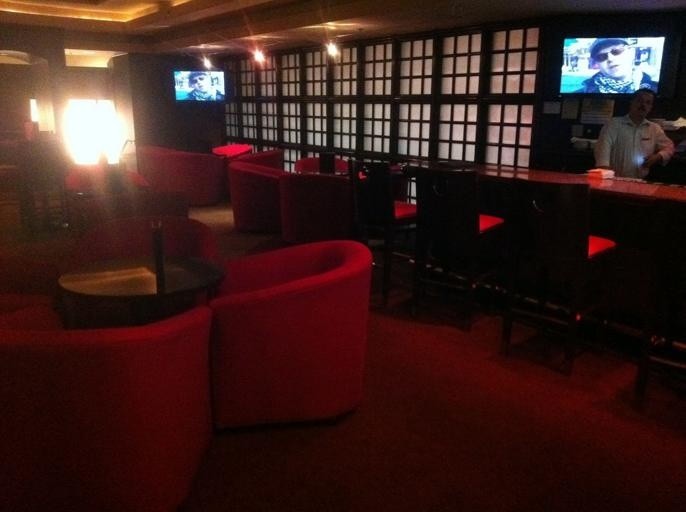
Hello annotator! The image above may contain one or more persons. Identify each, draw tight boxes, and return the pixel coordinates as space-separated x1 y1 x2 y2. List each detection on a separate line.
591 87 674 179
183 72 224 101
574 37 658 94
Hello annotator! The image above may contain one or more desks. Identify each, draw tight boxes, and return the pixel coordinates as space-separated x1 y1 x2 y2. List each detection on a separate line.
55 255 225 317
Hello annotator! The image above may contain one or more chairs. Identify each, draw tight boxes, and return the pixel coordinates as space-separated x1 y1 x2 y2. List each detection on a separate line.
0 301 215 512
206 235 376 431
401 165 497 331
493 175 620 375
69 216 224 262
136 145 399 246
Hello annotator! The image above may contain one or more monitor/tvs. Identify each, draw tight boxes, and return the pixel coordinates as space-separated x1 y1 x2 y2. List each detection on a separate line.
550 29 682 100
168 65 233 106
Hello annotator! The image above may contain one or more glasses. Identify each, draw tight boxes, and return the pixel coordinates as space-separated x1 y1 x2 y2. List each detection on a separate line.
190 77 203 84
594 45 628 62
